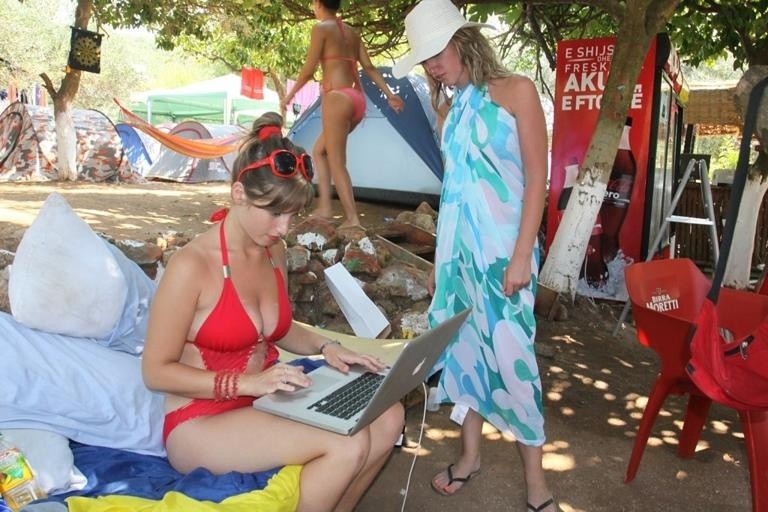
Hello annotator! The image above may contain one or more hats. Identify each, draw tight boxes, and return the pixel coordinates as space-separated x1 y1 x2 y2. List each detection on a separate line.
391 0 495 80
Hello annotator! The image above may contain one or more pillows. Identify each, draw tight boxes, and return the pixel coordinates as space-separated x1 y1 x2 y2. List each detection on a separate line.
6 189 159 343
0 425 89 500
1 308 167 458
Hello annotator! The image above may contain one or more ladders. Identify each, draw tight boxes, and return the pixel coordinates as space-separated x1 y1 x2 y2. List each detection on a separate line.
612 159 720 338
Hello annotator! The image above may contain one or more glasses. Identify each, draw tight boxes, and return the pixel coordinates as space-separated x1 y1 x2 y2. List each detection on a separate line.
236 149 314 181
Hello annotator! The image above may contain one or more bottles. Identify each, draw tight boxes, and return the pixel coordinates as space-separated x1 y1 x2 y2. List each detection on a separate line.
600 116 637 266
0 435 47 512
554 155 610 292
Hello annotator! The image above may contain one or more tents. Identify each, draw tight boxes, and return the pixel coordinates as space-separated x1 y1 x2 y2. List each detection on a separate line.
0 98 248 185
286 65 444 211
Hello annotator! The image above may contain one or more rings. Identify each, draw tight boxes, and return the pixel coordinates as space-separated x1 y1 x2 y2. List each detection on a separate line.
283 367 288 374
284 375 288 383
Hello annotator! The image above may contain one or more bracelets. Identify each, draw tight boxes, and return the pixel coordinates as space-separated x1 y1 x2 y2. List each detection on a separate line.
232 374 240 400
225 373 232 400
214 373 223 402
319 340 342 351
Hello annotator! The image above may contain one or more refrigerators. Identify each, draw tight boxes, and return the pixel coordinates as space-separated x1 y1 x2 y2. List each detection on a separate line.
546 32 691 300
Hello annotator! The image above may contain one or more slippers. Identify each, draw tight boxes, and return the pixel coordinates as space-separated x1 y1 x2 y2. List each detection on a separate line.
430 464 480 496
526 499 552 512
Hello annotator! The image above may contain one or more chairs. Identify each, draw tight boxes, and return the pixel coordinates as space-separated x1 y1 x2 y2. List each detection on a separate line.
621 257 768 511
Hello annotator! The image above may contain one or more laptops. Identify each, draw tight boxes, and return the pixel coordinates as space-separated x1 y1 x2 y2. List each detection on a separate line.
253 306 472 437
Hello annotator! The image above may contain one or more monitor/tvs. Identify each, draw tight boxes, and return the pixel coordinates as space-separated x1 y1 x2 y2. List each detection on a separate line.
680 154 710 180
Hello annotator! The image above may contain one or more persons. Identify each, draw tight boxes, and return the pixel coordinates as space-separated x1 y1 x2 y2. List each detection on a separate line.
142 112 405 512
281 1 404 229
389 0 560 512
